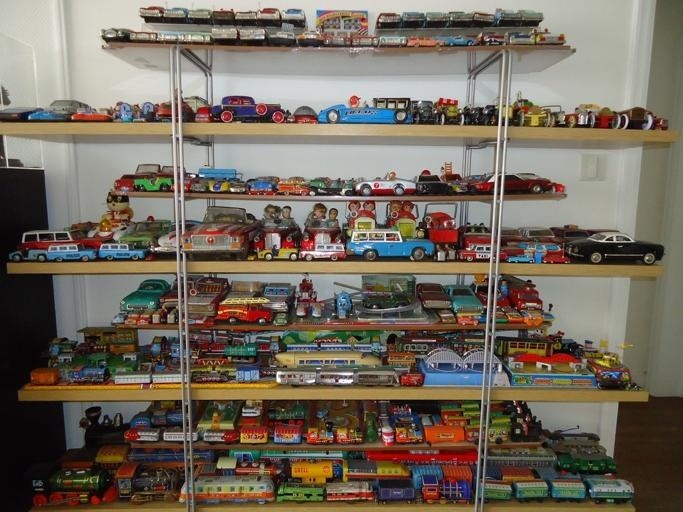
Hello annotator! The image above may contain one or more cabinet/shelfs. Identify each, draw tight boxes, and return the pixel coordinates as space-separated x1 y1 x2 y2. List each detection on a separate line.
3 31 679 512
